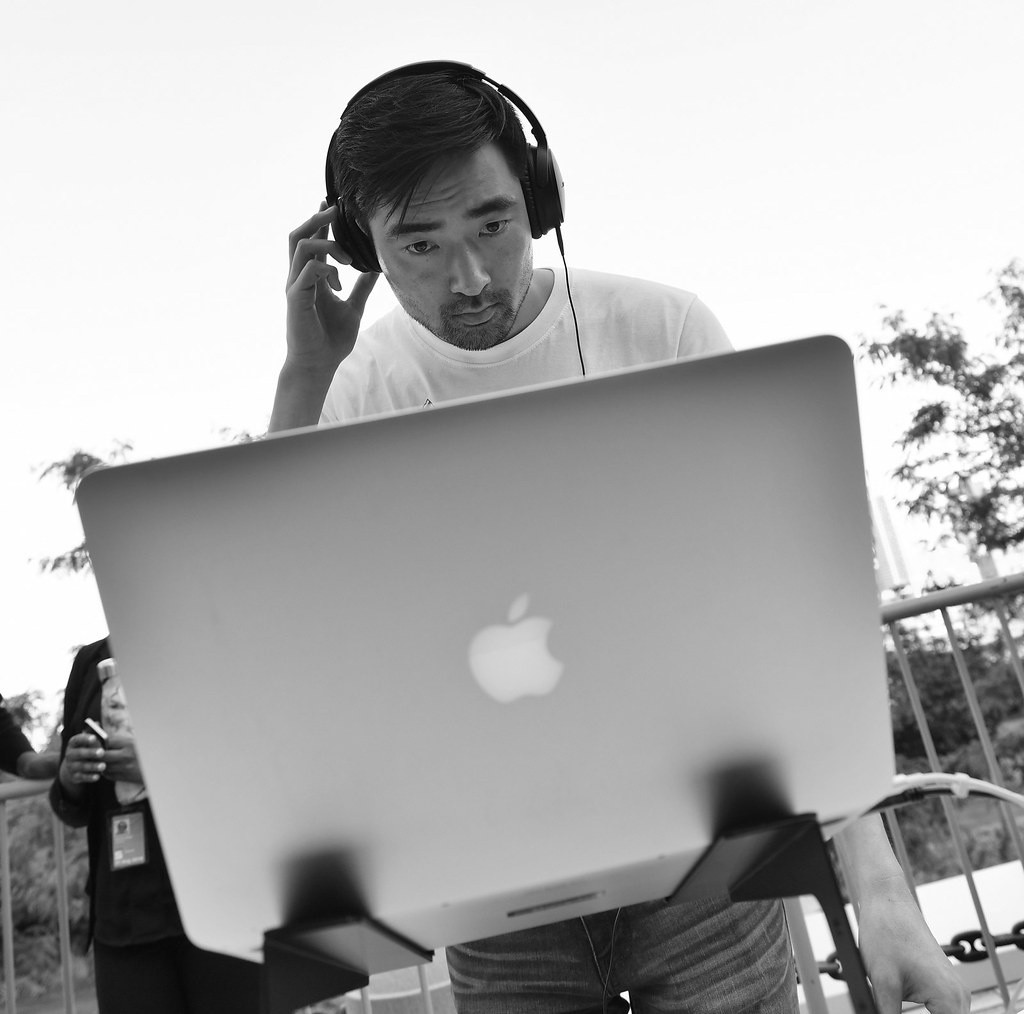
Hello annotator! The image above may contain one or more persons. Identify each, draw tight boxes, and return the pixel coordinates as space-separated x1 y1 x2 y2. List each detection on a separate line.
0 636 265 1014
270 60 972 1014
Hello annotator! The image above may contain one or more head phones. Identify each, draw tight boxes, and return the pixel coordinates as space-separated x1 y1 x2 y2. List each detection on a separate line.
325 58 563 271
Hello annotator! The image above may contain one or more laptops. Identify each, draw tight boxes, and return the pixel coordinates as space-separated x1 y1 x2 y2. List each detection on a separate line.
74 333 895 961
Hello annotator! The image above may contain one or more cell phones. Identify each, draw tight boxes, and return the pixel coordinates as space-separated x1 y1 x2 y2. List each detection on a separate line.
83 717 109 749
